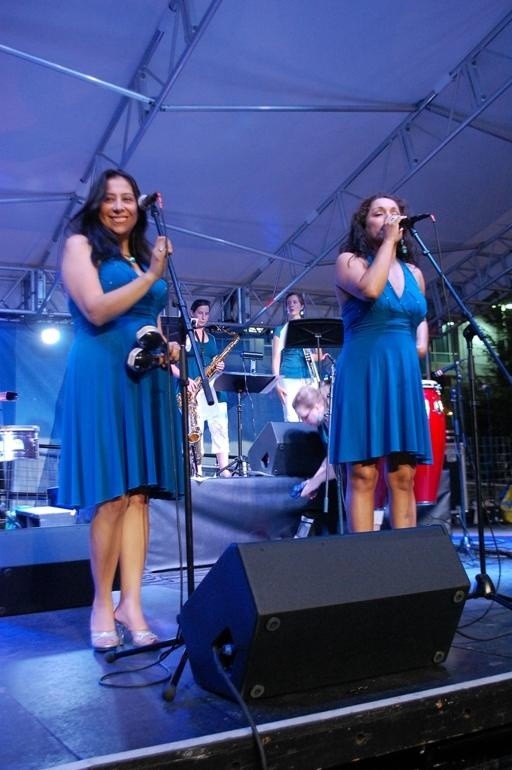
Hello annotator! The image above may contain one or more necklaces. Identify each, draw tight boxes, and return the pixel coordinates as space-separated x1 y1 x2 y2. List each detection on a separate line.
121 251 137 263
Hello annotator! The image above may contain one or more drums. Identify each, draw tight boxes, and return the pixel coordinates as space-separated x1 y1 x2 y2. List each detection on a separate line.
342 379 446 509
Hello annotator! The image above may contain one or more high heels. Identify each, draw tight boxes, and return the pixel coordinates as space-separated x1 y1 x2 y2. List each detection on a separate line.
90 621 159 651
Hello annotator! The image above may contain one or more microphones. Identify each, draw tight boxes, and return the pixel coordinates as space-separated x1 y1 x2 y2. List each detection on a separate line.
397 210 434 234
434 357 466 379
0 391 19 404
136 189 165 216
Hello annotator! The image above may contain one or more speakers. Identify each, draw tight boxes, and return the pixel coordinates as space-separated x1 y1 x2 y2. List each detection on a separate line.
247 418 328 477
0 522 109 618
175 522 471 705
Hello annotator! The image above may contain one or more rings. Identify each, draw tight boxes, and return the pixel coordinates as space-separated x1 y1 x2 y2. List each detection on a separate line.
158 246 163 252
390 217 397 221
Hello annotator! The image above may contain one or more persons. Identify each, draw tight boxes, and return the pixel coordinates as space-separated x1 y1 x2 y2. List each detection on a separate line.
326 189 430 534
170 299 234 480
291 385 342 502
53 166 187 653
271 292 324 423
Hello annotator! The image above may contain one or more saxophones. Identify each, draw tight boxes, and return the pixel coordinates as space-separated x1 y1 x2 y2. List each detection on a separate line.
176 325 240 443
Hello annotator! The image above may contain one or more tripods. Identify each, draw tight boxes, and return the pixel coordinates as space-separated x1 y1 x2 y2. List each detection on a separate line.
215 393 253 478
429 325 512 616
454 382 512 563
